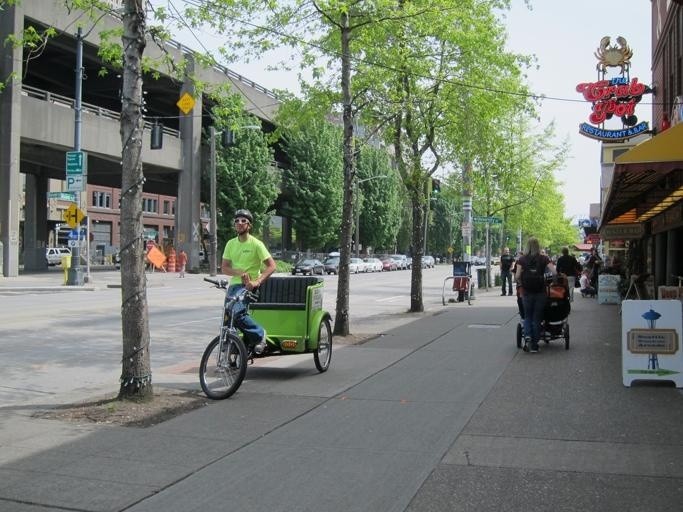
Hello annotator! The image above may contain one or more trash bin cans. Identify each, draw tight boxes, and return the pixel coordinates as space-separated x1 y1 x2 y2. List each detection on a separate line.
495 276 503 286
62 255 71 282
477 268 486 289
112 254 119 265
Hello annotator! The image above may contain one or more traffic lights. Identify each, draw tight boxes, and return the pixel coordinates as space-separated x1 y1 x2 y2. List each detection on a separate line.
433 179 440 192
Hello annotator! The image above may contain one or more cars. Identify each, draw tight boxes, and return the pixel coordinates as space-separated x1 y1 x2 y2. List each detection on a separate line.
470 256 501 266
291 255 434 276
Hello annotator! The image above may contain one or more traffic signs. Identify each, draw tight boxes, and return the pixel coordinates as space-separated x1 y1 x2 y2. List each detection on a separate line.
473 216 502 223
66 151 88 192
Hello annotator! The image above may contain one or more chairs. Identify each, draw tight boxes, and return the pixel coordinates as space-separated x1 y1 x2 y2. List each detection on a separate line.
250 276 325 336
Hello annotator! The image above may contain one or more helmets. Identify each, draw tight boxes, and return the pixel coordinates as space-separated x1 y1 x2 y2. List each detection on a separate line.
234 209 254 223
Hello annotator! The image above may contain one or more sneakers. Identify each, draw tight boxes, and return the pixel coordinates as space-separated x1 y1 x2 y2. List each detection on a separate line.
523 337 538 352
254 329 267 351
500 293 512 296
214 361 231 372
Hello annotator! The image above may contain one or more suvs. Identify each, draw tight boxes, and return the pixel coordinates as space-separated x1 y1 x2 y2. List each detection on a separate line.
45 248 71 265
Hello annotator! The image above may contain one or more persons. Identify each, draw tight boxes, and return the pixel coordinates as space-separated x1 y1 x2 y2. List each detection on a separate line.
177 249 187 278
500 247 619 298
213 209 277 374
514 237 557 354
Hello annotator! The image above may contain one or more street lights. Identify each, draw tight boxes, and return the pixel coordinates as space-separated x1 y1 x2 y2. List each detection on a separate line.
355 175 389 257
209 125 262 276
425 198 438 201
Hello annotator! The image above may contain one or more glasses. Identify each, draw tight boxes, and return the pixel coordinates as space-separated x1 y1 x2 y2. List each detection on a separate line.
235 219 249 224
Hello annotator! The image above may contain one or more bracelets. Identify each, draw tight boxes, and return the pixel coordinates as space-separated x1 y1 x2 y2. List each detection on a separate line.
255 278 260 288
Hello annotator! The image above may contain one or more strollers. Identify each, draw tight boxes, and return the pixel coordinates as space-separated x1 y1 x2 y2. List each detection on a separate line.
516 277 570 350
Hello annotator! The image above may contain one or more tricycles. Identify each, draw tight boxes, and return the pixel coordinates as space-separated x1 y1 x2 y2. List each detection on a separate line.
199 276 334 400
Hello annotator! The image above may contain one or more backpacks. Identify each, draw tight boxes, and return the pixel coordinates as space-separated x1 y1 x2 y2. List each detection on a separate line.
520 261 544 293
